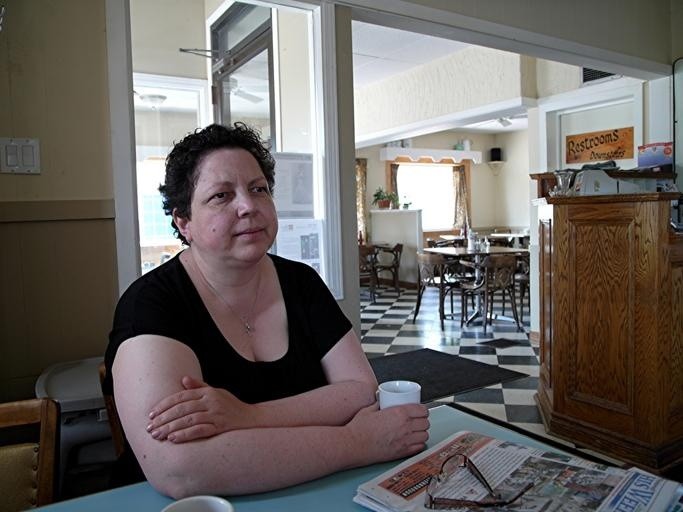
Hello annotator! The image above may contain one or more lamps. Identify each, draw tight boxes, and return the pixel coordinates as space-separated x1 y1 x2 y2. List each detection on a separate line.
138 94 167 110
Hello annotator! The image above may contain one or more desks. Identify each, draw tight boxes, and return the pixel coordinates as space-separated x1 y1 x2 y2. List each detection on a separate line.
29 399 683 512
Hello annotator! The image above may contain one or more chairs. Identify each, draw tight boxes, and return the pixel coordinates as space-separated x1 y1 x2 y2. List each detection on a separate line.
355 228 531 338
97 361 145 486
0 398 61 512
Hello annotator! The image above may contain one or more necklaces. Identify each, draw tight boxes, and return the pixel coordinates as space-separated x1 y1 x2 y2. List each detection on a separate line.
192 262 262 336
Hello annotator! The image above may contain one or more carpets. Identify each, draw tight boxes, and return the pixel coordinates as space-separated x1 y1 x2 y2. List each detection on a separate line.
367 348 533 405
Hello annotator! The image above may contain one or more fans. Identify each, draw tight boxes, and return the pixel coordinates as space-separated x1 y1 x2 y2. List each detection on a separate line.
229 77 264 104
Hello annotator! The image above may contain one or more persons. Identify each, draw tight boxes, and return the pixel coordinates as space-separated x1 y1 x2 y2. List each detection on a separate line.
103 121 430 502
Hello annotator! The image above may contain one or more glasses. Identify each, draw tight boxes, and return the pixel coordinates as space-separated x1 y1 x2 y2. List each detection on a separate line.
424 453 534 510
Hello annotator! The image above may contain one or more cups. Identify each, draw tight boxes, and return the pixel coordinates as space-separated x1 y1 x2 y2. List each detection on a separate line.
553 171 574 196
374 380 422 413
159 495 232 512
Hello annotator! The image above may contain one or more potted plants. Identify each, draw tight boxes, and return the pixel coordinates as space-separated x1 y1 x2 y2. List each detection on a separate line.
371 186 400 209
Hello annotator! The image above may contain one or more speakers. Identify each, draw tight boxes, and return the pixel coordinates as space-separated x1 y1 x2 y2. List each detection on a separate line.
490 147 502 160
